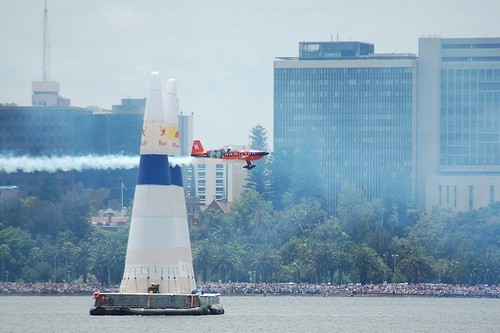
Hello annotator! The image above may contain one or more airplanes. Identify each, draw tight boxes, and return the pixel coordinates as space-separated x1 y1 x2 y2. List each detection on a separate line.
190 141 269 171
